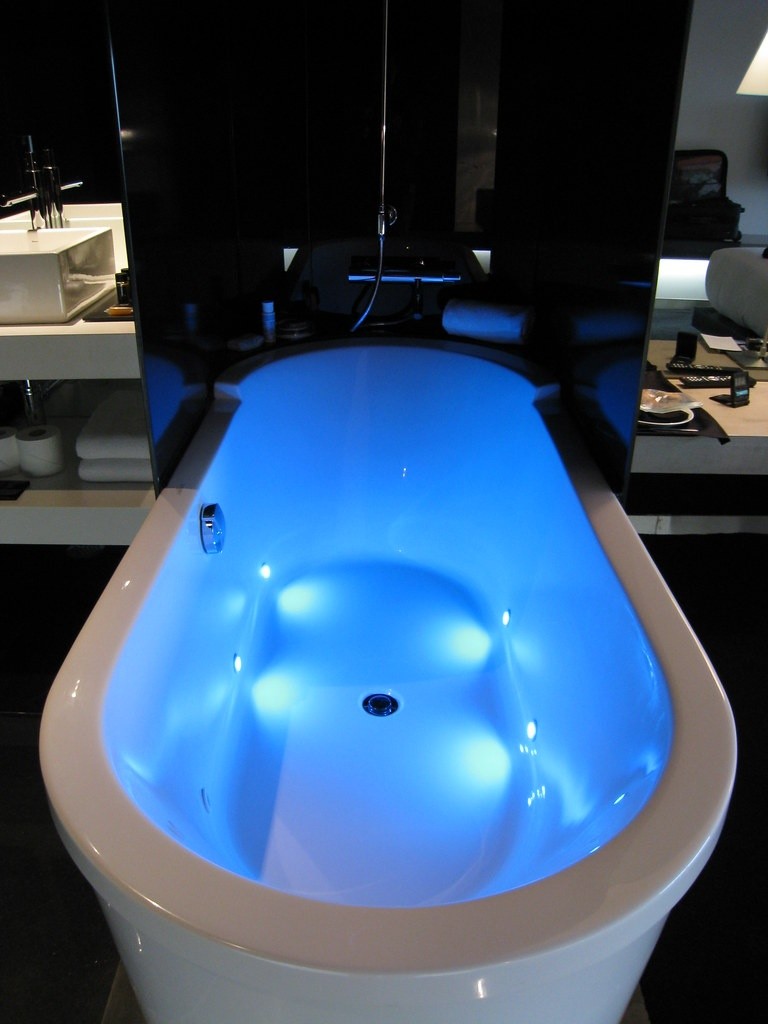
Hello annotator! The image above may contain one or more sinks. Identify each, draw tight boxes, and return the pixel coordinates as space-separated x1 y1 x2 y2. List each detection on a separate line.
0 203 128 273
0 228 116 324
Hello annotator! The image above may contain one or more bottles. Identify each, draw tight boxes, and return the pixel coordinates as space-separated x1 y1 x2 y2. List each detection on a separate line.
115 268 130 304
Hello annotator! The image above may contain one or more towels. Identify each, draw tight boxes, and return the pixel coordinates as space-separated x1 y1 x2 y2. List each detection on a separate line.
76 390 155 482
442 300 525 343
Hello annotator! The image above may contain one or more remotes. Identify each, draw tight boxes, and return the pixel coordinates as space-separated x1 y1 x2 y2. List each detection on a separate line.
680 375 756 389
665 362 743 375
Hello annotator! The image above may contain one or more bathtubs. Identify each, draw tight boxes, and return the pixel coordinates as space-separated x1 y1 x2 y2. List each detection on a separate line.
39 336 738 1024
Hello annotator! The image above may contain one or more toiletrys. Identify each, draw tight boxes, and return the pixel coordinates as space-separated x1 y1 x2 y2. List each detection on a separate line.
263 302 275 339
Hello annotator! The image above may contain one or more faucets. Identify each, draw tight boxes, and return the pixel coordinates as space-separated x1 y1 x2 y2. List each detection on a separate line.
0 162 66 228
30 161 83 226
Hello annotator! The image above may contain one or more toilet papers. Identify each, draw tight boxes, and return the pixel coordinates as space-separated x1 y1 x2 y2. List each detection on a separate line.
0 426 65 476
